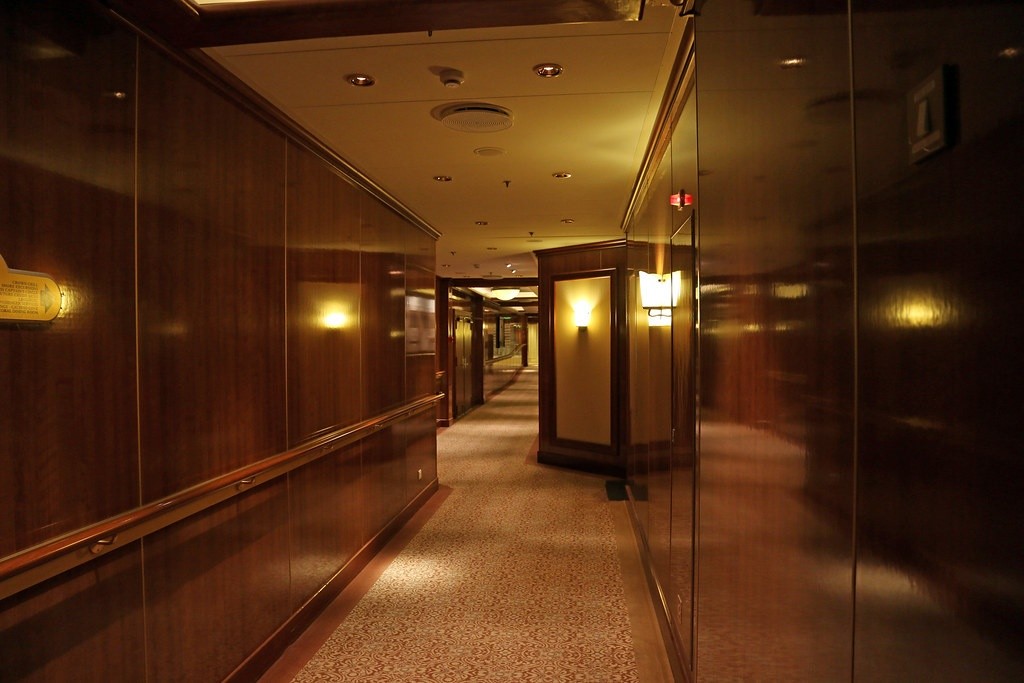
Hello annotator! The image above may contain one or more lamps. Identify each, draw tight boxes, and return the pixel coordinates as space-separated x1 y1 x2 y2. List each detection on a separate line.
638 270 662 317
